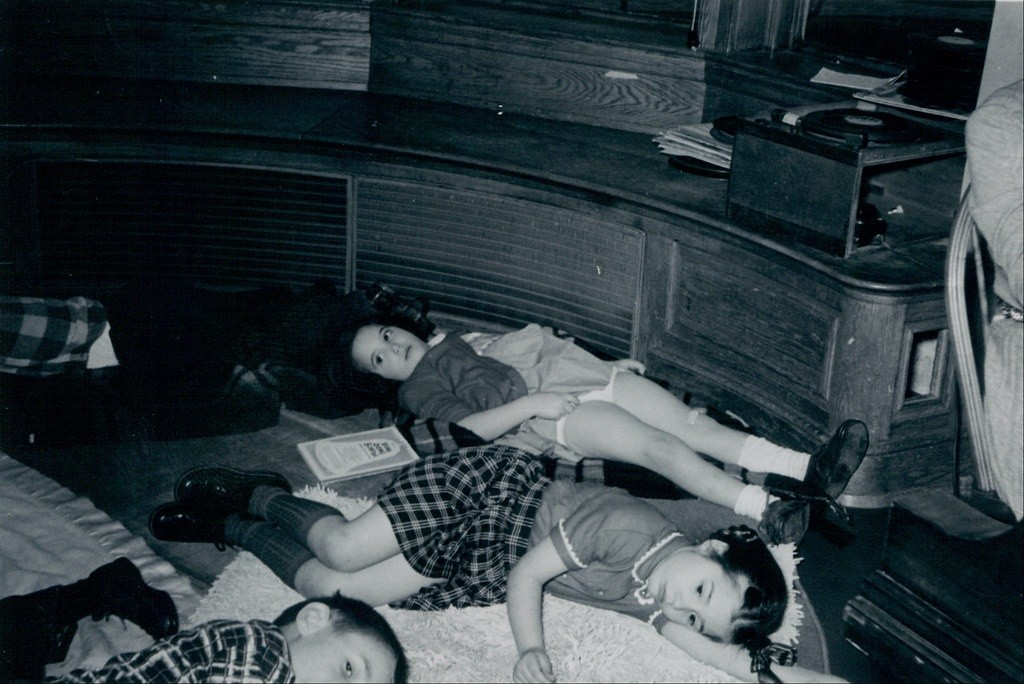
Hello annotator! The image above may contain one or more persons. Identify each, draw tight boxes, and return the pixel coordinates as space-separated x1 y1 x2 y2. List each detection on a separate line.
962 75 1024 522
318 300 869 546
47 588 413 684
145 445 849 684
0 556 180 684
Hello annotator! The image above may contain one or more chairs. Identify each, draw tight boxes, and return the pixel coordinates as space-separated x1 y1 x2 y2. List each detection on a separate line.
944 178 996 501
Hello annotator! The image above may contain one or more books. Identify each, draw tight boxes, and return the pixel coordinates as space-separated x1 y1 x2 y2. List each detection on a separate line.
654 118 736 172
297 424 421 486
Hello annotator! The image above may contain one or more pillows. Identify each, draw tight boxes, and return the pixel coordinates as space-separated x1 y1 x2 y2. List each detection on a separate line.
0 295 107 377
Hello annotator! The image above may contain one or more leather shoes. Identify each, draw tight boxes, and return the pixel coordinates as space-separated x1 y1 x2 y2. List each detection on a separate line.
148 501 239 553
174 466 292 526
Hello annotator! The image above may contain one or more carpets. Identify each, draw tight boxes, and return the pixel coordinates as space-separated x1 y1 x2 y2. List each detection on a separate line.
179 485 803 681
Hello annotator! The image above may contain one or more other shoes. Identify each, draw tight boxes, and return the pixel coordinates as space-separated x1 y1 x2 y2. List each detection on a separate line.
759 494 812 545
803 419 870 502
86 555 180 638
